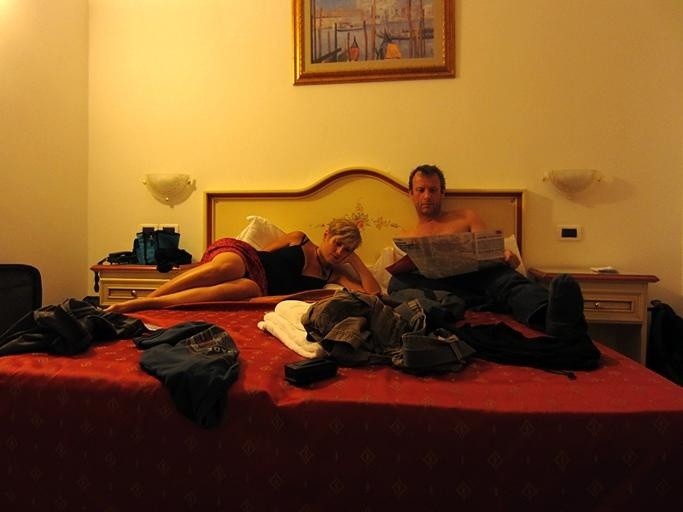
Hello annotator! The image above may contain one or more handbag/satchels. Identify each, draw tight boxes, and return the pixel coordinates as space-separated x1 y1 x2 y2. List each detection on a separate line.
135 225 181 263
647 299 683 385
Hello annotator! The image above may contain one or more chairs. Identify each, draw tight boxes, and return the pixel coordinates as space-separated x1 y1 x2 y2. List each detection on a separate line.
0 265 42 339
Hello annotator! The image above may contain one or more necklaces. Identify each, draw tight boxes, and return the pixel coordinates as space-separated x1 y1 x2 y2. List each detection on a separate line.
314 247 327 277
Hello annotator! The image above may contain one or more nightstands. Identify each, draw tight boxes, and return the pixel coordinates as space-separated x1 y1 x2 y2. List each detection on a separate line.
529 265 659 367
90 263 195 311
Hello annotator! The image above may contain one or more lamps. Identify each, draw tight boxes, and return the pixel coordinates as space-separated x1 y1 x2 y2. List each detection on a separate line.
541 169 603 199
140 172 191 201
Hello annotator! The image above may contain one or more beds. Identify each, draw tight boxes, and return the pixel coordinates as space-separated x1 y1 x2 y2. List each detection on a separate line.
0 166 683 511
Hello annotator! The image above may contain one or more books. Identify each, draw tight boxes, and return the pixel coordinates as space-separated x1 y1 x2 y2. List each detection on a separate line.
589 266 619 272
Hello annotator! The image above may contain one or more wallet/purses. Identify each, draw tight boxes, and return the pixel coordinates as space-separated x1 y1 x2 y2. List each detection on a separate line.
284 355 338 385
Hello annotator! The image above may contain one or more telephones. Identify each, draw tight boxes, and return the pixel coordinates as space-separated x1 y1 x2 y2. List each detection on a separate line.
107 250 139 265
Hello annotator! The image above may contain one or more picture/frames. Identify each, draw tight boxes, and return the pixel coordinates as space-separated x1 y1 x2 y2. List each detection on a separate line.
292 0 456 87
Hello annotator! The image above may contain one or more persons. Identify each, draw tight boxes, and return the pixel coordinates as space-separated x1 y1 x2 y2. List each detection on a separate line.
384 162 585 339
102 218 381 317
